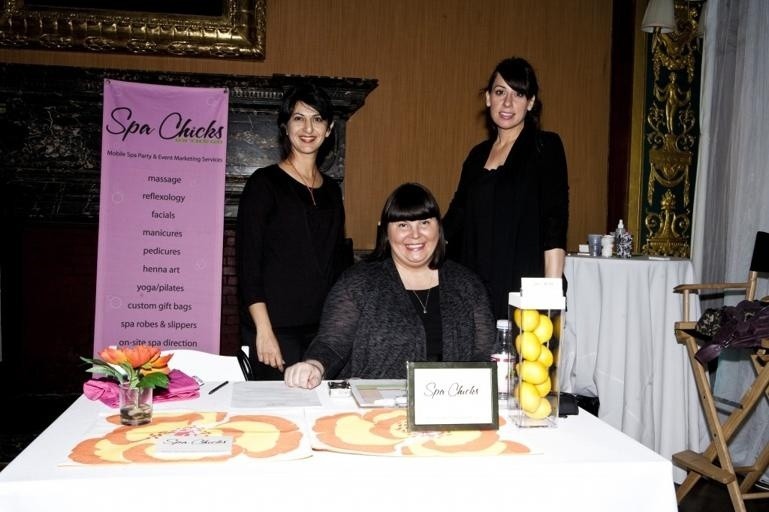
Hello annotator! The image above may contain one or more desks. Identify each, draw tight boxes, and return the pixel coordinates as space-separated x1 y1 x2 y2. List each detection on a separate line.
559 257 710 485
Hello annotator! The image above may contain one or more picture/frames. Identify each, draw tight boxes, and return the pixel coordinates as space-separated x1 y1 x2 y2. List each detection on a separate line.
0 0 266 62
406 362 497 431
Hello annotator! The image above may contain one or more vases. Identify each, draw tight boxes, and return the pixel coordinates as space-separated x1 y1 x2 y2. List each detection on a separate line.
119 387 152 425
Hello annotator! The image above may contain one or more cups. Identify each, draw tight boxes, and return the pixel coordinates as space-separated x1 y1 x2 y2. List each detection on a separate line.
120 383 154 426
588 234 602 256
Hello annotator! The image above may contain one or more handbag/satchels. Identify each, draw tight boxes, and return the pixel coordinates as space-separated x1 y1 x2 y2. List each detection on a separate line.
692 298 769 365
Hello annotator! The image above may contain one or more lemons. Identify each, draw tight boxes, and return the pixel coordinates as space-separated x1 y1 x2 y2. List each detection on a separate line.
526 398 551 419
517 360 547 384
520 332 540 360
521 310 539 332
538 343 553 368
535 376 551 397
513 308 523 331
519 382 539 412
534 313 553 342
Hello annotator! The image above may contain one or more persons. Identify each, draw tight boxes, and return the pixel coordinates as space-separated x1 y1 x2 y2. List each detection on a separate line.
284 183 495 390
438 56 569 334
235 84 344 380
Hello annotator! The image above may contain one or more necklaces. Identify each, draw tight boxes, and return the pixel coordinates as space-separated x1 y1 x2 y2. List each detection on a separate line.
397 266 434 313
287 159 318 205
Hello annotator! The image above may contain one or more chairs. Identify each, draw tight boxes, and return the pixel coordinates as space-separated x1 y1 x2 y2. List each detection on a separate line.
672 232 769 512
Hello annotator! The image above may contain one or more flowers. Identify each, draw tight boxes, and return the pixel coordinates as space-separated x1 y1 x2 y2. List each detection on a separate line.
80 345 174 388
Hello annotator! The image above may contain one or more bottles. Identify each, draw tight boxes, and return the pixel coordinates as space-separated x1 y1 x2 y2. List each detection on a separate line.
615 219 626 257
490 320 516 399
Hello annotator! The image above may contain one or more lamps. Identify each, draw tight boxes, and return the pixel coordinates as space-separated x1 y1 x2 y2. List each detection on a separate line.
642 0 681 57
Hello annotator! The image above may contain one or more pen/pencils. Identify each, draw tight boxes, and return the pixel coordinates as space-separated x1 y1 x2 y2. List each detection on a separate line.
209 381 230 395
